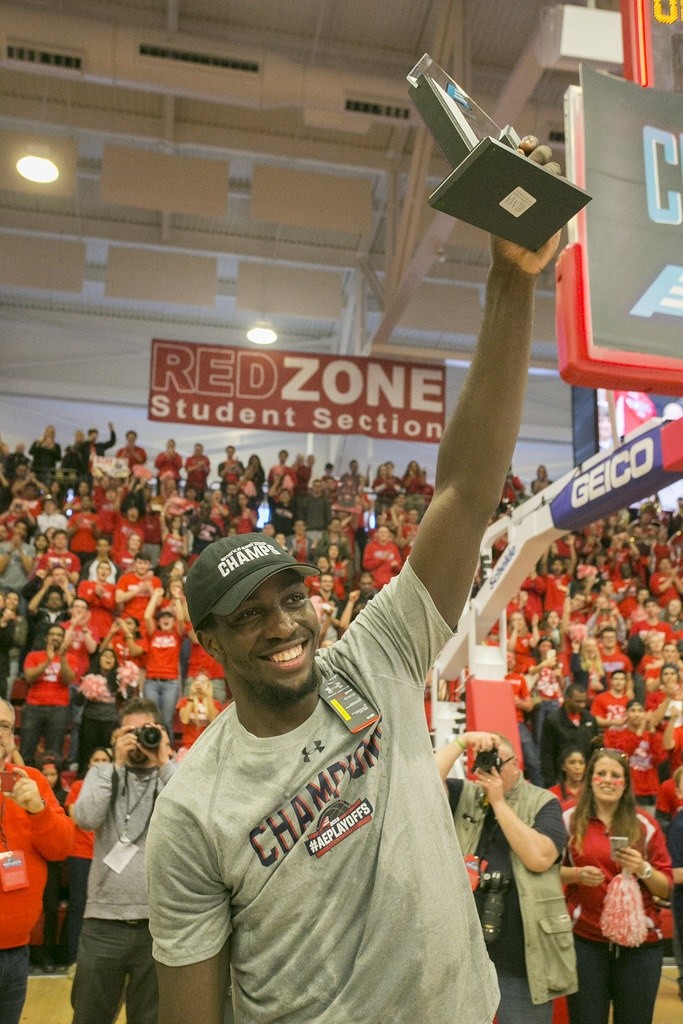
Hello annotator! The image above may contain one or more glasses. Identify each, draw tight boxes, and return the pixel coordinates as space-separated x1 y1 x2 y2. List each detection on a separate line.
0 721 15 734
591 749 628 762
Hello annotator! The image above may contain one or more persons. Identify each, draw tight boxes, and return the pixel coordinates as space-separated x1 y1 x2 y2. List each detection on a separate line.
0 423 683 974
71 698 176 1024
143 133 569 1024
0 700 74 1024
598 388 683 511
666 766 683 1003
436 729 578 1024
562 748 674 1024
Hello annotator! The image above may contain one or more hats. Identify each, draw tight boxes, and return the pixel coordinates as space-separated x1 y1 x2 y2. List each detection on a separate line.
185 533 322 629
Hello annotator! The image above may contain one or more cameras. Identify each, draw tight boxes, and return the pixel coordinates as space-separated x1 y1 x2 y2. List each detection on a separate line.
471 747 502 776
128 726 161 750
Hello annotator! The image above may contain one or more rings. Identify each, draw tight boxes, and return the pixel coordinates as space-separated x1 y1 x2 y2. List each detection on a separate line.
488 779 491 781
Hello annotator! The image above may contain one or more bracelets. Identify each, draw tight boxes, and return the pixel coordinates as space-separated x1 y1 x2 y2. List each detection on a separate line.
456 737 466 750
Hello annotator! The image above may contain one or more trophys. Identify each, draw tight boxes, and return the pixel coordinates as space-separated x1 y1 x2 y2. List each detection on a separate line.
406 52 594 254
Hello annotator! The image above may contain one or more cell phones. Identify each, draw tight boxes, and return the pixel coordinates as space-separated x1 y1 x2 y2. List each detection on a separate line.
0 771 20 792
609 837 628 861
546 649 556 660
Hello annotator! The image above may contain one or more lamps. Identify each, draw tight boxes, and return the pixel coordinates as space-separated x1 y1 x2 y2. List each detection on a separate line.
247 310 277 344
14 144 59 183
538 0 624 74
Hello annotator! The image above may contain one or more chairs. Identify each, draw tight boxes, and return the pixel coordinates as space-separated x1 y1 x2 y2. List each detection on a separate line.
172 709 183 751
10 677 69 761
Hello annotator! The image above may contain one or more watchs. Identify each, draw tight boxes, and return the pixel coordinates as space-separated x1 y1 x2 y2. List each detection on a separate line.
639 862 651 879
